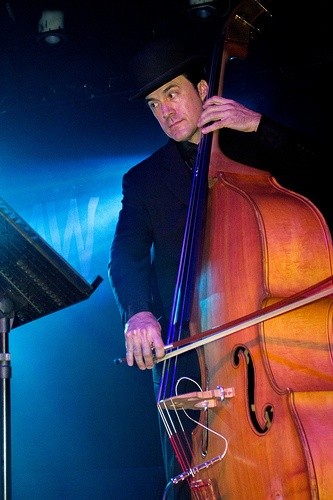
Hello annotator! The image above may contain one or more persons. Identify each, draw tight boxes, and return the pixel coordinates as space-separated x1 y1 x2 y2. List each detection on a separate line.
109 43 305 496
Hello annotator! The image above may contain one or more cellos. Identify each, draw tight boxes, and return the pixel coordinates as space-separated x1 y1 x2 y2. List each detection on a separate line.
152 0 332 500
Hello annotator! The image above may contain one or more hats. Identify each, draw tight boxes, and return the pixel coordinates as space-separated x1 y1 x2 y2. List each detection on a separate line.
128 37 193 100
